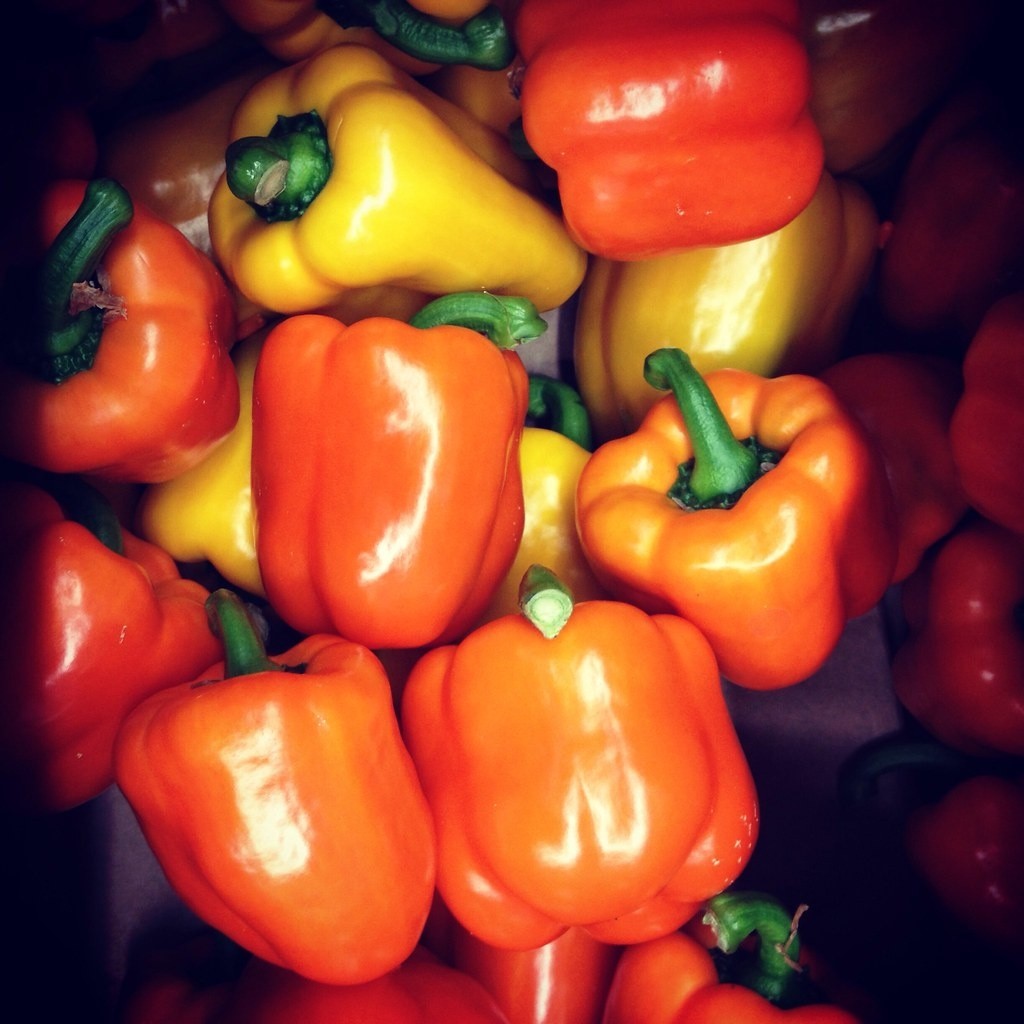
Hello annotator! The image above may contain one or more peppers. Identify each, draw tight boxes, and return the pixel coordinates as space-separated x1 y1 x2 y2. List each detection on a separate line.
0 0 1024 1024
